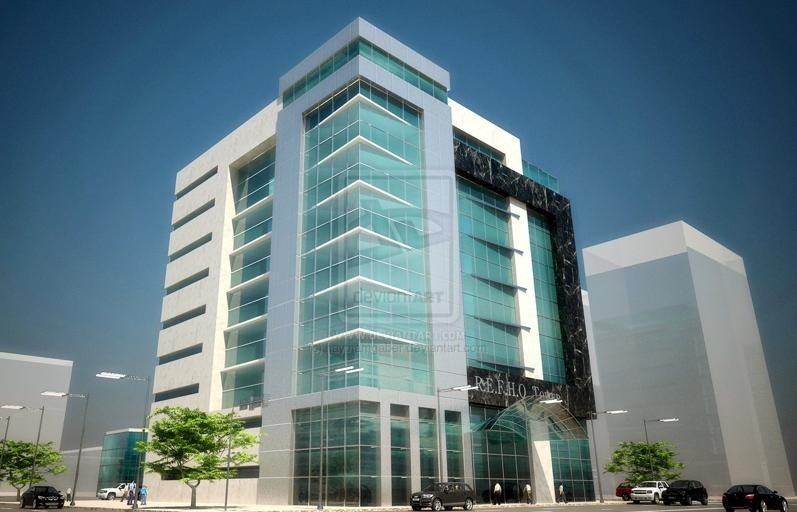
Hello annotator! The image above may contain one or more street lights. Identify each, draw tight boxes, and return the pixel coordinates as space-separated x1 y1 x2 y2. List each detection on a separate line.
318 365 355 509
96 372 150 508
2 405 45 488
438 386 479 481
41 390 89 506
644 418 680 481
590 410 627 503
325 368 364 404
526 399 563 503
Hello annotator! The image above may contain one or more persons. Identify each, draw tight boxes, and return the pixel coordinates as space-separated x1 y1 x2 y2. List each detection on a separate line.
119 481 128 502
139 485 147 505
554 479 568 504
66 486 72 501
522 481 534 504
490 480 501 505
126 481 135 506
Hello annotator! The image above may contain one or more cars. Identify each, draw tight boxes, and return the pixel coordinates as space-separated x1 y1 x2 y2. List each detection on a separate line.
20 486 65 509
722 485 788 512
616 479 669 505
410 483 476 511
98 483 136 500
662 480 708 506
